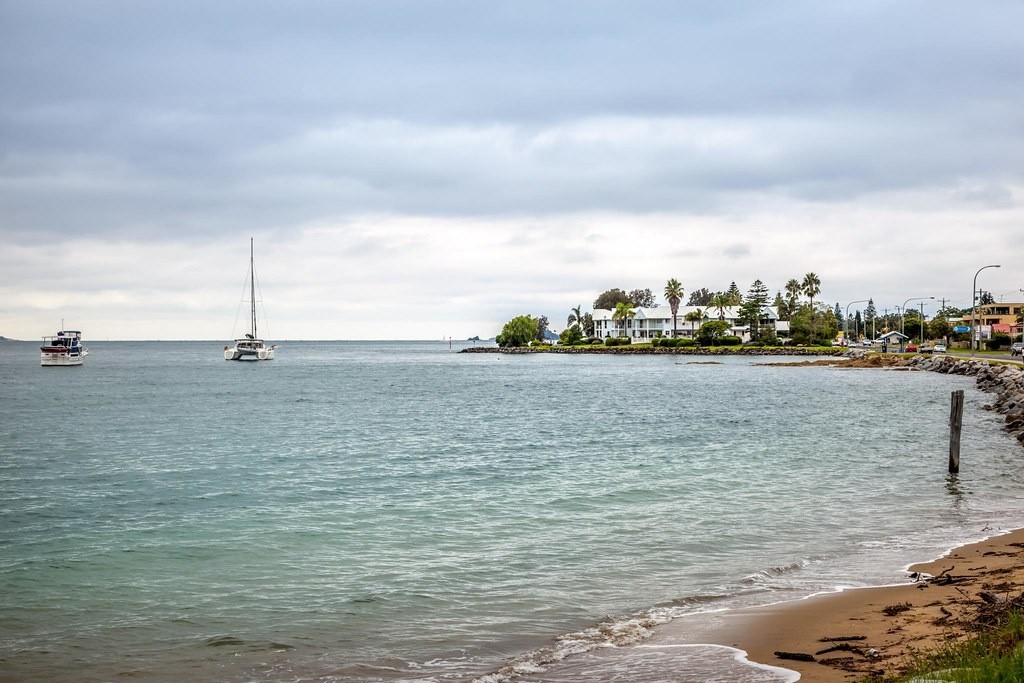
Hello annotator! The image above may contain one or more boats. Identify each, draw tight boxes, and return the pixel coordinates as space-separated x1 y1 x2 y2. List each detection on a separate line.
39 319 83 366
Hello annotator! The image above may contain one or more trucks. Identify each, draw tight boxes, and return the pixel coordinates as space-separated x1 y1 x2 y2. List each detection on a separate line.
835 330 855 341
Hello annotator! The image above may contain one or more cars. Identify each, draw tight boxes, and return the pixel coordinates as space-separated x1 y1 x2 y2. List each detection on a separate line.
1010 343 1024 356
848 341 857 348
905 345 917 352
863 340 871 346
934 345 946 353
832 338 847 347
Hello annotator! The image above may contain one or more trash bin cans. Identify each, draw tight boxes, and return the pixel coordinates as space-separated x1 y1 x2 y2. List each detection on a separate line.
881 341 887 352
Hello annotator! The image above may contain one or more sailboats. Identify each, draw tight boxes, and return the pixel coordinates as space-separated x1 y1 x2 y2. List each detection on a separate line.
223 237 275 361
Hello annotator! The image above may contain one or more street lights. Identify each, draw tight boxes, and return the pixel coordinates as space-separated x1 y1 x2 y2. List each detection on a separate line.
847 300 870 347
901 297 934 353
972 265 1001 355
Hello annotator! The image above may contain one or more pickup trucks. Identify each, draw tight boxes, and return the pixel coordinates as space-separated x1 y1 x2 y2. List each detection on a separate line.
917 343 933 354
